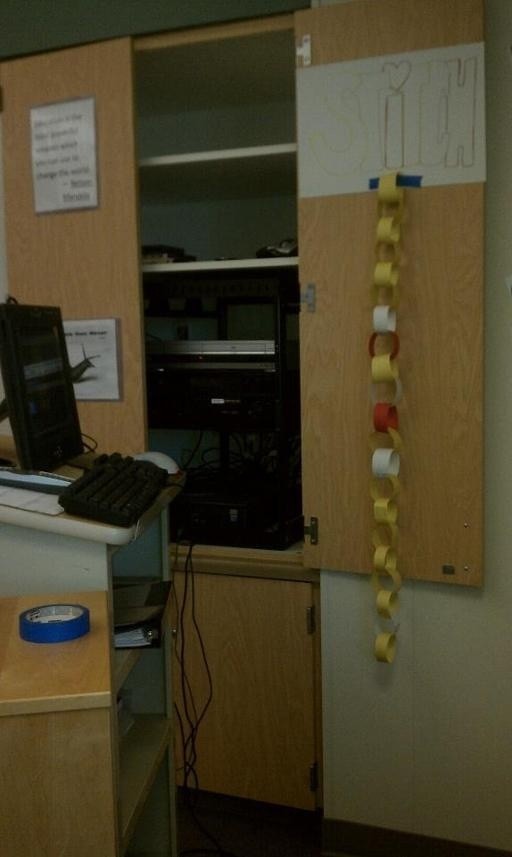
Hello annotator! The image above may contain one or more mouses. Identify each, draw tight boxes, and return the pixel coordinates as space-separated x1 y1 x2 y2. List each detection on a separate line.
131 452 180 475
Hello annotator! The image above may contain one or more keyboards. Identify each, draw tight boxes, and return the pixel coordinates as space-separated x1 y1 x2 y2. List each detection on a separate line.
59 451 168 526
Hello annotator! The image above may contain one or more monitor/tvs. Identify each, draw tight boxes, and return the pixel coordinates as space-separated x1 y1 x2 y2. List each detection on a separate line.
0 304 84 474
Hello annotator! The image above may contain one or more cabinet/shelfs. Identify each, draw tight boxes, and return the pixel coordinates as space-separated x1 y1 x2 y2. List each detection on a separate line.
108 501 171 854
170 570 317 814
0 1 490 589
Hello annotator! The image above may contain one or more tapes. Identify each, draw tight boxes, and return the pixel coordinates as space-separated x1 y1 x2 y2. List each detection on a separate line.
20 604 89 642
